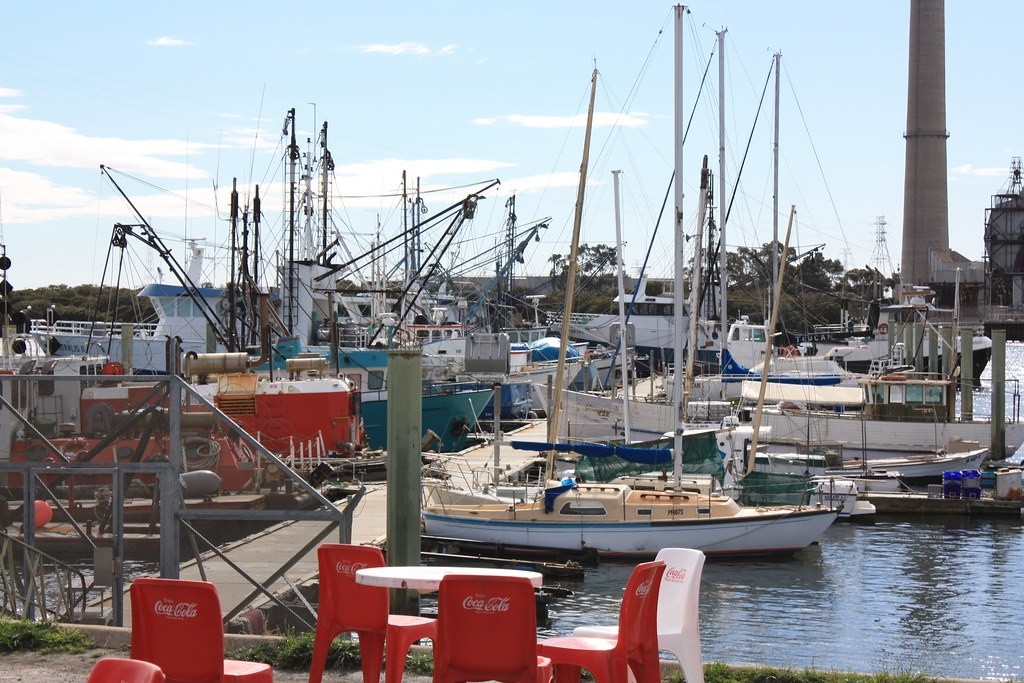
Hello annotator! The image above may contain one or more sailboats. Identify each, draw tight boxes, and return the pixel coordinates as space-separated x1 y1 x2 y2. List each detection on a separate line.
0 1 1024 561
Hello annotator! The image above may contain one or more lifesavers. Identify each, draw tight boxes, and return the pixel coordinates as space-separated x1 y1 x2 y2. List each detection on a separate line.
881 376 907 381
60 438 90 461
878 323 888 336
783 345 798 359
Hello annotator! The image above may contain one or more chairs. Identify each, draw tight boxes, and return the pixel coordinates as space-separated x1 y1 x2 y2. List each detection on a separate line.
573 547 706 683
307 543 438 683
86 578 273 683
537 560 666 683
433 574 553 683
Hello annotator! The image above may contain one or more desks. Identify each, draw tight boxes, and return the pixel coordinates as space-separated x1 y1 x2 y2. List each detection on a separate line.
355 566 544 588
